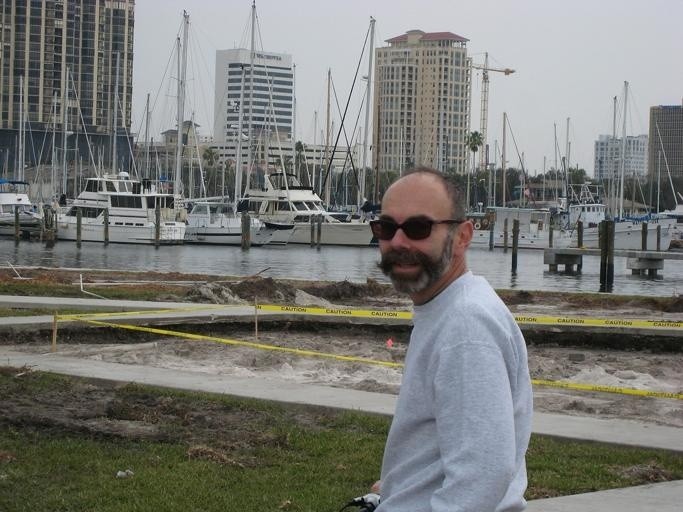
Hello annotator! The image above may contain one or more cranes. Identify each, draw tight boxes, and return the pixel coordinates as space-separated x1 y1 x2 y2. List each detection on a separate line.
470 50 517 185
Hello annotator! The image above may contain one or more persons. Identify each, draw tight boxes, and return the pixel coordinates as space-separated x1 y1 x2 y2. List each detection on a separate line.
370 168 534 512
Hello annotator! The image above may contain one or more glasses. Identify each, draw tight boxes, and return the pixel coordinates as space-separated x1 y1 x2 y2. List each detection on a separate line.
368 215 465 242
